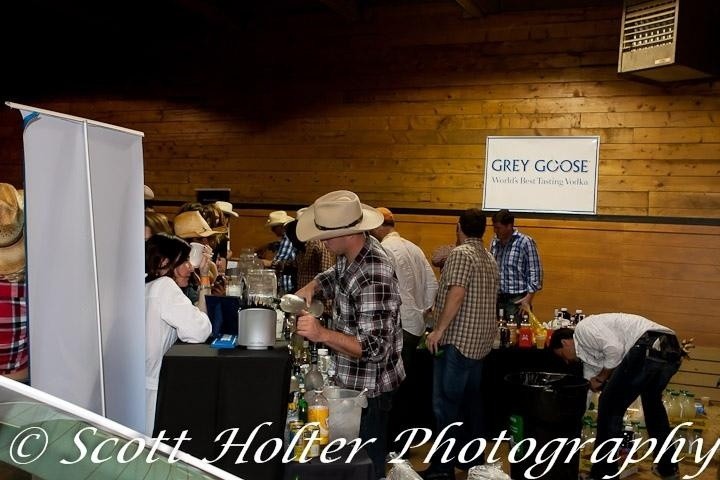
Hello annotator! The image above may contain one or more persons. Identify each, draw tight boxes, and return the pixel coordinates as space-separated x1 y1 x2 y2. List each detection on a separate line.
174 210 226 296
415 208 501 480
261 210 296 295
143 210 212 307
176 203 216 228
549 312 682 480
369 206 440 460
285 220 335 349
211 201 239 264
145 232 212 440
293 190 406 480
489 209 544 323
141 185 155 210
0 183 30 383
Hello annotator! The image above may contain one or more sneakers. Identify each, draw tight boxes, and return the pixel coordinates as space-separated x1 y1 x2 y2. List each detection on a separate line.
651 463 681 479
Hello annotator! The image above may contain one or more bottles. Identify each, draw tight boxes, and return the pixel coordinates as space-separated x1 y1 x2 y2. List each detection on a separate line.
299 389 308 422
497 321 509 348
304 364 324 389
311 343 317 363
676 429 689 453
301 342 313 377
557 307 571 328
323 370 338 387
497 308 507 326
304 424 320 458
583 409 598 437
423 331 446 357
684 393 696 422
291 425 305 462
622 426 633 451
519 315 532 349
535 320 547 349
695 400 703 425
571 309 585 327
271 294 324 318
292 392 300 420
318 349 331 372
289 421 304 440
309 388 330 446
669 390 682 426
693 430 702 454
288 344 304 402
663 389 670 420
492 316 501 349
506 314 516 346
547 321 553 348
631 421 640 442
552 309 562 329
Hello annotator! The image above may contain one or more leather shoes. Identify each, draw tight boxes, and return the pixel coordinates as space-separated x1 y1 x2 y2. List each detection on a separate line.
415 464 457 480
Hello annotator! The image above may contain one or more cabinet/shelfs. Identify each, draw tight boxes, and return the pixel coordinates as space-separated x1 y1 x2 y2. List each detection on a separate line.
165 333 374 480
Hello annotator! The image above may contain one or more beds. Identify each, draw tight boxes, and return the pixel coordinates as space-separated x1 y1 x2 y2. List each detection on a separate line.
478 345 588 434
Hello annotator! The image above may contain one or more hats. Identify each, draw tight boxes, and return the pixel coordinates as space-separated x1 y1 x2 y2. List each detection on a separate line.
143 183 154 200
376 206 394 222
265 210 291 226
173 210 228 238
216 200 239 218
0 182 26 274
295 189 383 241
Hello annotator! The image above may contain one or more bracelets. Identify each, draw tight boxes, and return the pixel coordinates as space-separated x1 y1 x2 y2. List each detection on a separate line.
199 276 209 277
